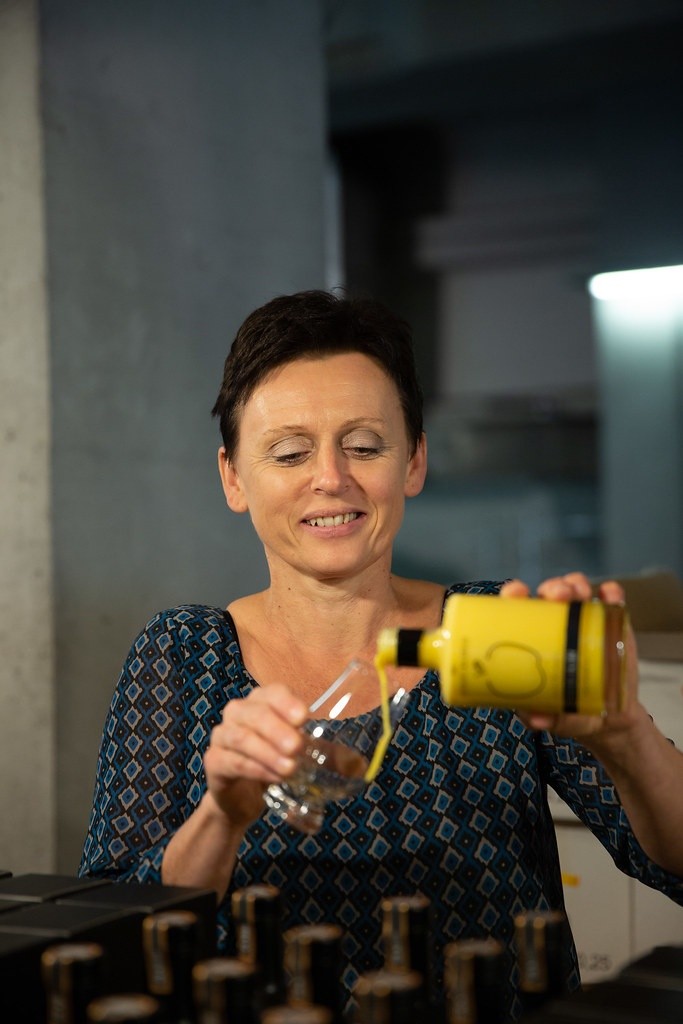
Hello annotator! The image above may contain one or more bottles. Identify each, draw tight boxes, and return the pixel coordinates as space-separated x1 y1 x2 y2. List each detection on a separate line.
38 882 567 1024
378 593 627 719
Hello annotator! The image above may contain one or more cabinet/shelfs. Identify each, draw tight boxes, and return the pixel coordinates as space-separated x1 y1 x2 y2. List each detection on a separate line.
533 660 683 983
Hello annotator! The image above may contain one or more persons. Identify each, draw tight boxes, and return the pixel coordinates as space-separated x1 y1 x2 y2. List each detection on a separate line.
78 288 683 986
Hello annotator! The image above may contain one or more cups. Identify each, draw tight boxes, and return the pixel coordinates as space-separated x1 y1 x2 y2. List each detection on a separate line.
260 658 409 834
